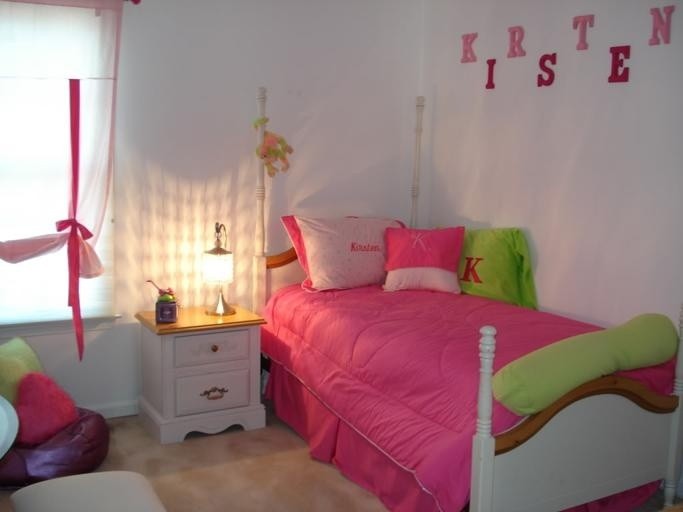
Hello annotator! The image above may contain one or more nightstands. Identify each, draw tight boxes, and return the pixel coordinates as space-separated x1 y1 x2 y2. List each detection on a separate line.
135 305 267 445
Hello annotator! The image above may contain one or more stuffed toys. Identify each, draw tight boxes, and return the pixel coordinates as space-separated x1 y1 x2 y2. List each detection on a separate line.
251 117 295 176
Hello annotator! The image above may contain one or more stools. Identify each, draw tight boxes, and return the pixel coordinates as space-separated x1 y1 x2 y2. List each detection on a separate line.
0 470 169 512
0 408 109 486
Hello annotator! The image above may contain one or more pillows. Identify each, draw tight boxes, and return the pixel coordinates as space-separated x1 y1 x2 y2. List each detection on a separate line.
15 372 80 449
381 227 466 294
488 314 679 416
0 336 46 408
458 227 542 308
281 212 406 294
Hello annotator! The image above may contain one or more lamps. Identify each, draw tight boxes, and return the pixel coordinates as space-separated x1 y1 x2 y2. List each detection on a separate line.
203 221 235 317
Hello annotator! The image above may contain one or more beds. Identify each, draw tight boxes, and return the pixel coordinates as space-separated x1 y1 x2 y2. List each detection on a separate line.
254 86 683 512
